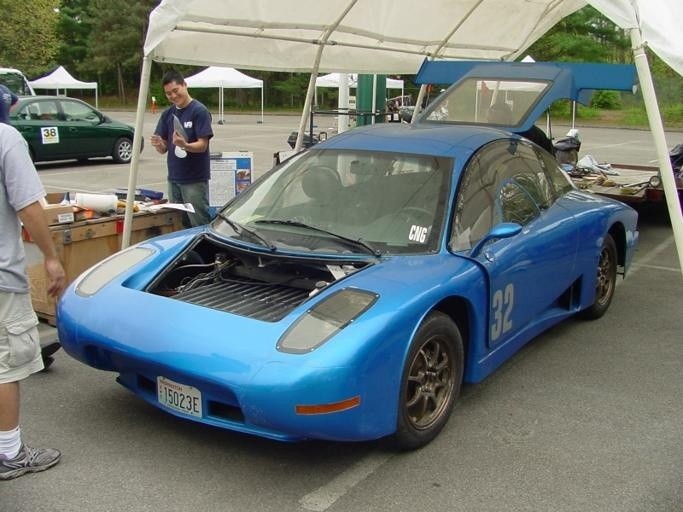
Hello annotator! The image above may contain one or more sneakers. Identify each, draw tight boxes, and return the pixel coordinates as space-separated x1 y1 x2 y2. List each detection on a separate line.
0 443 61 479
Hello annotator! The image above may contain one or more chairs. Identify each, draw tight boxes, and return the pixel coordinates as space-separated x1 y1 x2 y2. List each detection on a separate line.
299 168 370 226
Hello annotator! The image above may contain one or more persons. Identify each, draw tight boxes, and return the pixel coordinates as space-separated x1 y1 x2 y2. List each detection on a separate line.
148 69 213 228
0 82 66 481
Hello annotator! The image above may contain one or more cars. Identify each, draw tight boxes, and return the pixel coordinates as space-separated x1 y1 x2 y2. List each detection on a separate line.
6 96 144 166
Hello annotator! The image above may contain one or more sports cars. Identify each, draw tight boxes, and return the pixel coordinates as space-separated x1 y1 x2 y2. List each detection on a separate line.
51 57 642 451
